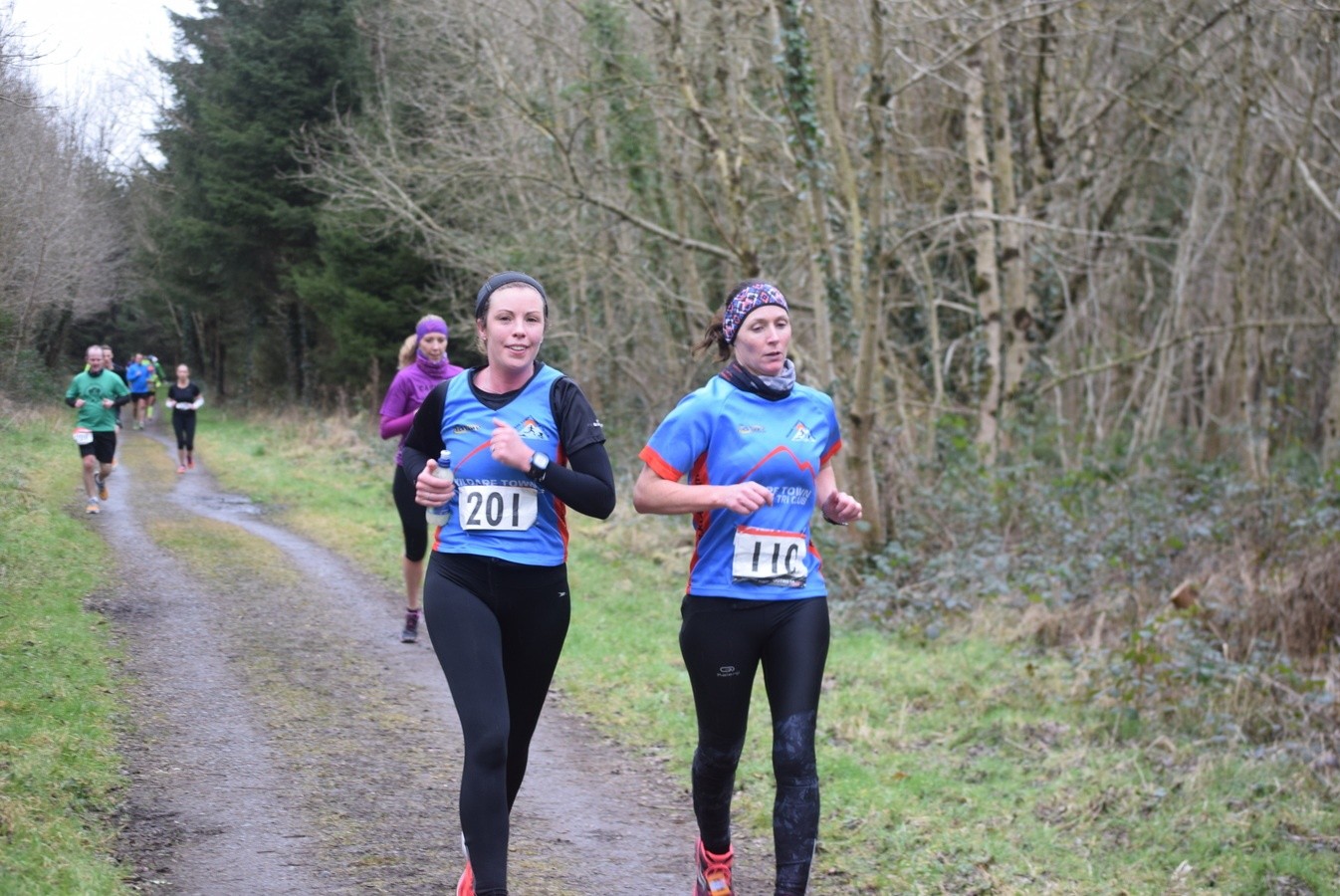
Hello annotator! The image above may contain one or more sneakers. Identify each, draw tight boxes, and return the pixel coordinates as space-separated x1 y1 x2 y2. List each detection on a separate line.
178 464 185 473
94 471 109 499
87 499 101 514
186 454 194 468
695 836 735 896
456 831 477 896
403 613 420 641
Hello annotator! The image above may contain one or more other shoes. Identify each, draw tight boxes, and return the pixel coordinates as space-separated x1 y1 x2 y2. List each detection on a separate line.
132 420 144 430
147 406 154 418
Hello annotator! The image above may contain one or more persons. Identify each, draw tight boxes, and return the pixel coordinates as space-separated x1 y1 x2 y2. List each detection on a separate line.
379 313 464 643
165 364 205 474
64 344 205 515
405 270 616 896
64 344 132 515
633 279 863 896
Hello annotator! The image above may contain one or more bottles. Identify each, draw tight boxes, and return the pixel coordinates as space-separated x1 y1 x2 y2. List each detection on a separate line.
425 450 454 526
